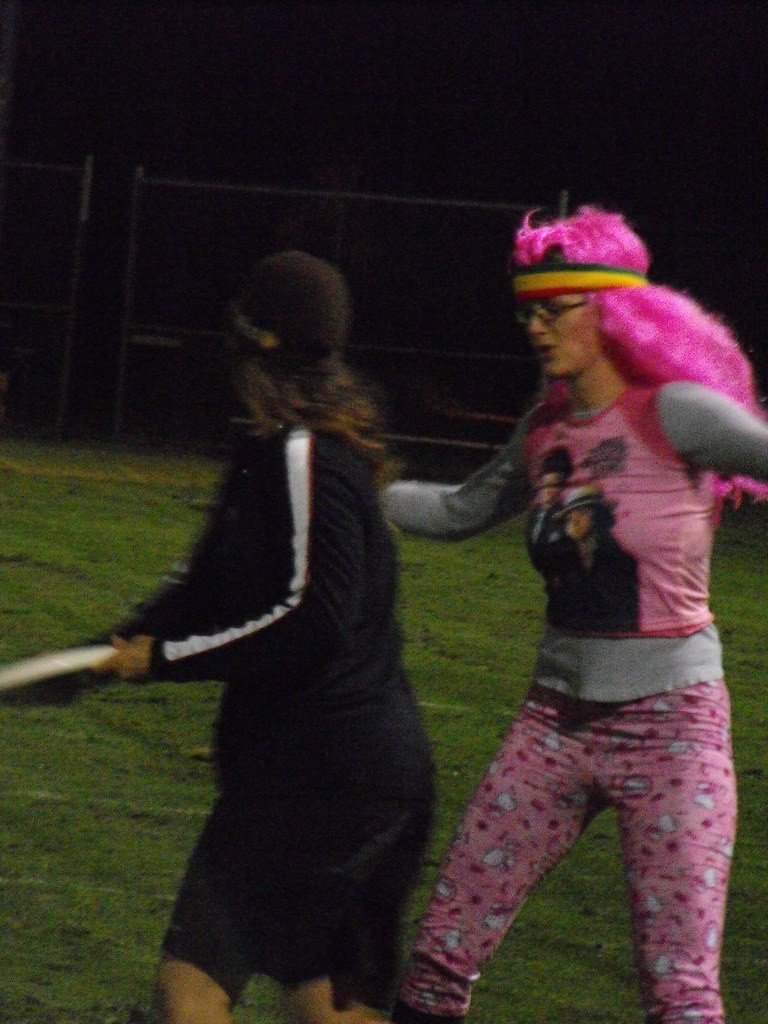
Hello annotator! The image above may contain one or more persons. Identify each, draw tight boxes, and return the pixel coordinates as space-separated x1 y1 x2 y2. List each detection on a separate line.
380 206 767 1024
0 249 437 1023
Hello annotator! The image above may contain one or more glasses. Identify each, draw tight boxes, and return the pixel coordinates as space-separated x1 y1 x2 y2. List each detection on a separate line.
514 296 593 328
